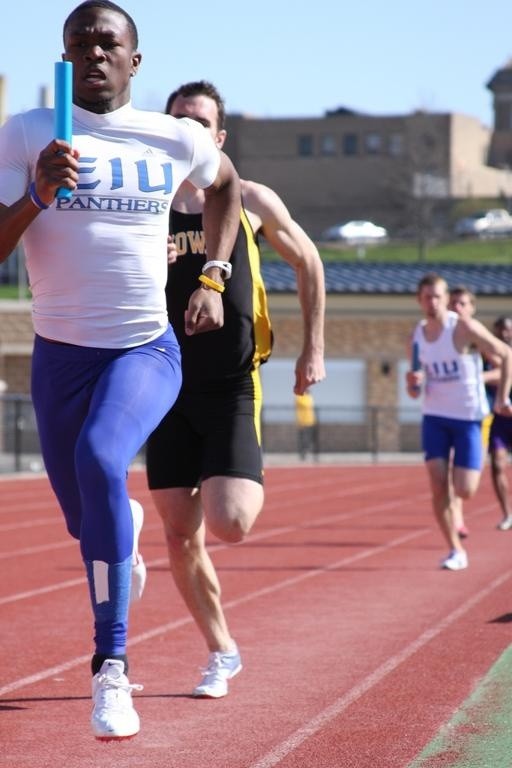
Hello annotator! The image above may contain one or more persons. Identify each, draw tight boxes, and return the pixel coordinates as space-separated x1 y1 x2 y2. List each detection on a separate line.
293 391 320 458
143 79 326 701
0 0 244 742
407 272 512 569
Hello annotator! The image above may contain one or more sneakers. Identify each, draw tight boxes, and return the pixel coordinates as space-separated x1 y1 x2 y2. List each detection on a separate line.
192 639 244 700
86 653 147 743
495 513 512 531
439 547 469 571
127 498 147 604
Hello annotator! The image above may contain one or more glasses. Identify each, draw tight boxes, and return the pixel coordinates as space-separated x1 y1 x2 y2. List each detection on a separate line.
451 300 473 305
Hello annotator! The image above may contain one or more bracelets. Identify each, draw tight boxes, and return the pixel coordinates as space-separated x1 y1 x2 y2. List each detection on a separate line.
197 273 227 293
200 260 234 279
27 181 49 211
